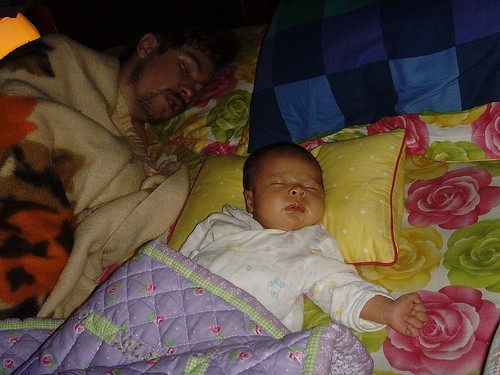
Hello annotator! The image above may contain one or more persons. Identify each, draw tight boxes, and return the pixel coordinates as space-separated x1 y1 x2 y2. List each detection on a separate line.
178 142 427 338
0 16 238 314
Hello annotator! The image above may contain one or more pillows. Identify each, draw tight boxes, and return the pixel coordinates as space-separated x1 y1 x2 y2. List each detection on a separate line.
106 20 265 157
166 129 404 266
245 0 500 152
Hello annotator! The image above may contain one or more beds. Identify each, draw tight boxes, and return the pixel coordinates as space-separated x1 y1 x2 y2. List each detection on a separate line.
108 104 499 374
